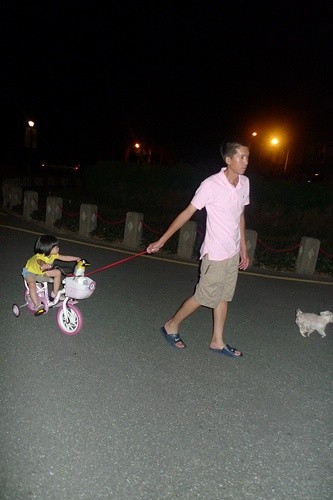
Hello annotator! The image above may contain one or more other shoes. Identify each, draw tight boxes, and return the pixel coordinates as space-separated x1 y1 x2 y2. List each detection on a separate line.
34 303 44 312
49 291 65 301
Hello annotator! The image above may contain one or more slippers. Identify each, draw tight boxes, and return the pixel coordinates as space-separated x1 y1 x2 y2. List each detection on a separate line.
209 345 242 358
160 327 186 349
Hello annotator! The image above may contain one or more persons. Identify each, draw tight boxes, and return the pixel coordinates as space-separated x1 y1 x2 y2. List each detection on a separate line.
22 234 85 312
147 139 251 358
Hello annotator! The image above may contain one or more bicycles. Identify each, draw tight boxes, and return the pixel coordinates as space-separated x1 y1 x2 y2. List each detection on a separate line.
12 259 95 336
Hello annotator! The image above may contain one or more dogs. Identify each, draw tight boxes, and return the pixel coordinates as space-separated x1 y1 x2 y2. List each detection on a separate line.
295 309 333 339
